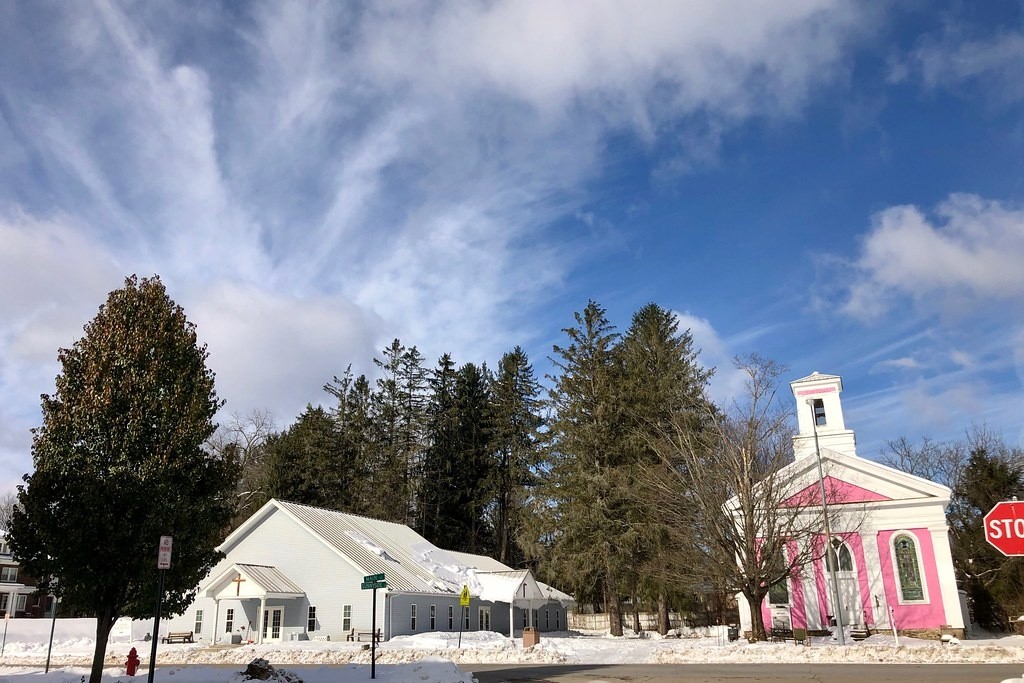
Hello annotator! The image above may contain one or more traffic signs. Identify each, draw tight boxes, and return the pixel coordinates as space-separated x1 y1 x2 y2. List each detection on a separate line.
361 574 385 582
361 581 387 590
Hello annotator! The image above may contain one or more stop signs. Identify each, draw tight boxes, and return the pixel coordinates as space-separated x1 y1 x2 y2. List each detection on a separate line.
983 500 1024 557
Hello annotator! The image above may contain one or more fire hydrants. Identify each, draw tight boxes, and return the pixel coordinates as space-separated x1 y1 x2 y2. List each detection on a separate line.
125 647 141 676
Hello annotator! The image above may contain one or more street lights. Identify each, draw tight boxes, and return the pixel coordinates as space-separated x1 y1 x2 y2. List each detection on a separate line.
806 398 845 647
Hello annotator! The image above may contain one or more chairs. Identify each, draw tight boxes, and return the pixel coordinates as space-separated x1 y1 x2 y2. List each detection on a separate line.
939 625 954 645
793 628 810 646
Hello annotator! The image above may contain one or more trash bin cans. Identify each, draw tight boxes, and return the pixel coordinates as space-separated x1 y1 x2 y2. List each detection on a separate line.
728 626 738 642
523 627 540 647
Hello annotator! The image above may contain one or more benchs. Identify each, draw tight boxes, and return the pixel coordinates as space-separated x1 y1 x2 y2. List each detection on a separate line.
347 628 381 643
162 631 193 644
770 628 792 643
